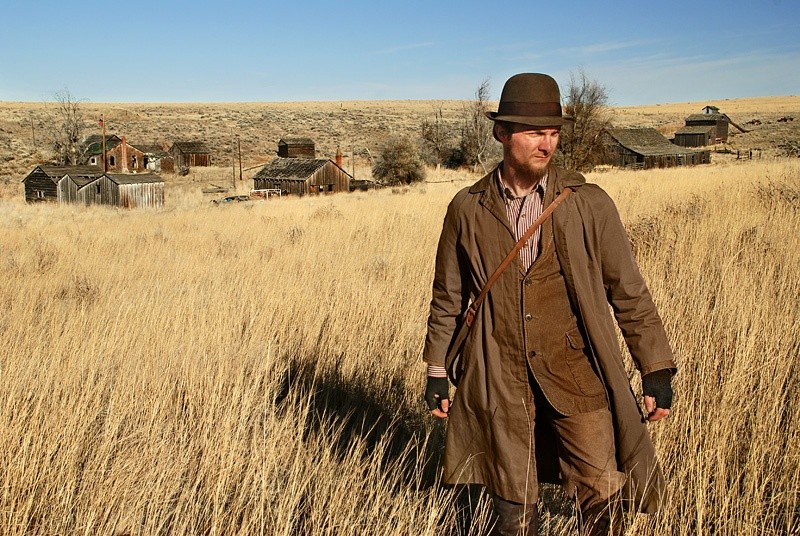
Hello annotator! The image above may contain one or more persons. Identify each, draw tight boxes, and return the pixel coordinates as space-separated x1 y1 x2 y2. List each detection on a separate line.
422 72 679 536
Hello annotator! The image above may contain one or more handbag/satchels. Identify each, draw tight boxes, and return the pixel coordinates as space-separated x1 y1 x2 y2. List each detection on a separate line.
444 308 478 387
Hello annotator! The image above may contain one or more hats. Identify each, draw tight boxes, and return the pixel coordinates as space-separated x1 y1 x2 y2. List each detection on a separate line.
486 73 576 127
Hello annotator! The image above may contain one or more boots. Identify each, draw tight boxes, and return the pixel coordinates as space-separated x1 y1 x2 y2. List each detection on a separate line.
492 492 538 536
579 491 625 536
